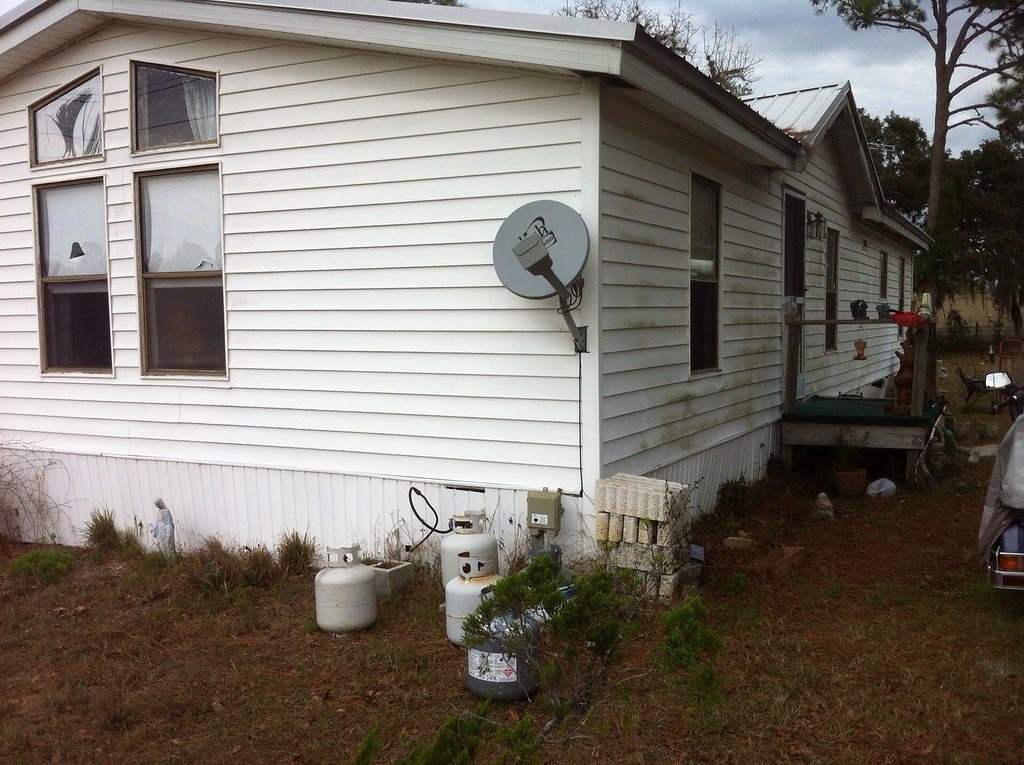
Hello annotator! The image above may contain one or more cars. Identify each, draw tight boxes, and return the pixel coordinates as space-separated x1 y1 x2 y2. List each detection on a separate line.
982 414 1024 596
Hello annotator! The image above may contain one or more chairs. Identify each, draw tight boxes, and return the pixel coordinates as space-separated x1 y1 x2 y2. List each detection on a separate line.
955 366 996 414
998 338 1024 413
